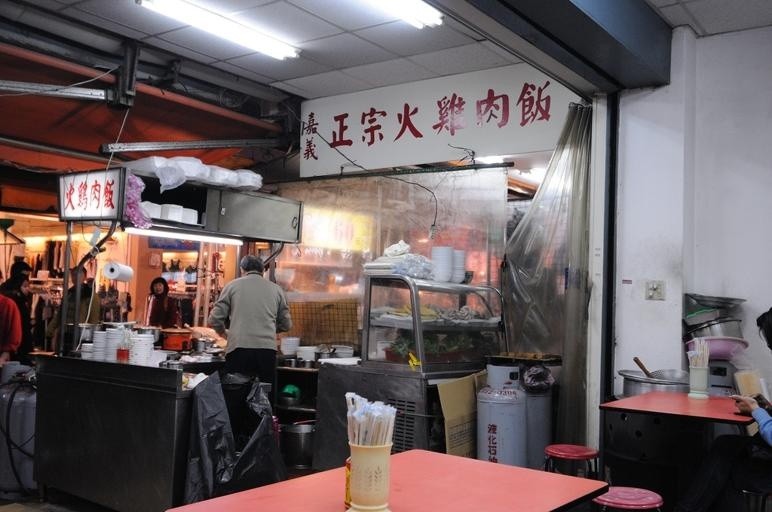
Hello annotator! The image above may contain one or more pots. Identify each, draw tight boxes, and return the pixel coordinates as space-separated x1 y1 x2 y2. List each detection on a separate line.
314 352 336 368
163 325 192 351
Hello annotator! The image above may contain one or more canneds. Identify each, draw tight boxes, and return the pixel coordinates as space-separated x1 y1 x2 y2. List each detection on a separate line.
116 348 129 363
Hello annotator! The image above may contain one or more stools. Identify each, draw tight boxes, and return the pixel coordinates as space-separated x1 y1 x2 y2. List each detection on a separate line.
736 469 772 509
542 445 663 511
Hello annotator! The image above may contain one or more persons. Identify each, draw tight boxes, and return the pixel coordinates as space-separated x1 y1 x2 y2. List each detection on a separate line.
207 255 291 418
144 276 182 328
46 267 101 339
0 260 34 368
712 307 772 489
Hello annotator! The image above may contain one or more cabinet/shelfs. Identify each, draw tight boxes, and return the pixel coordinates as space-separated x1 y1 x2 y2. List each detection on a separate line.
359 272 506 379
273 361 318 422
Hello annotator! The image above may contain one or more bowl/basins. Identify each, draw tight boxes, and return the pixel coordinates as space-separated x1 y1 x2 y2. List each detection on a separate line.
81 328 154 368
432 246 467 285
685 336 749 359
280 335 316 360
618 369 692 398
332 345 354 357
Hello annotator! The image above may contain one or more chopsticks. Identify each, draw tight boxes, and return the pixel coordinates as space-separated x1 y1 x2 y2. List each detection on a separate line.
345 393 396 445
